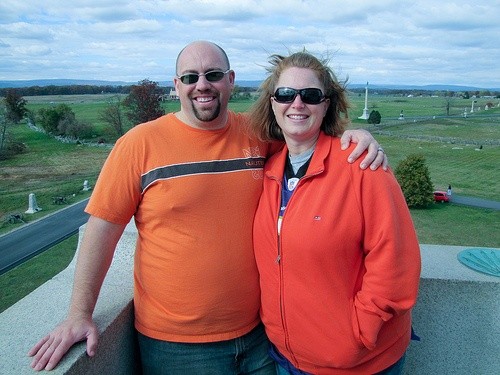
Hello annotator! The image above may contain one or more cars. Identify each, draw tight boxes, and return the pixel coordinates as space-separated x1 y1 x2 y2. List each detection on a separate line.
432 190 449 202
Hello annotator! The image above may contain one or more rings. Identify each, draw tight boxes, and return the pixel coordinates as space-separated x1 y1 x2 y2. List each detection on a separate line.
377 147 384 153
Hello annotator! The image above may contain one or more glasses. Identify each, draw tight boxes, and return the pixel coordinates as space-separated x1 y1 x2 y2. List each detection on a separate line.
270 87 326 105
176 70 230 84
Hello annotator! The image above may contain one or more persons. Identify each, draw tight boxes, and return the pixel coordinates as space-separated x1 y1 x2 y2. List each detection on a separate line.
448 183 452 196
28 40 389 375
247 53 422 375
